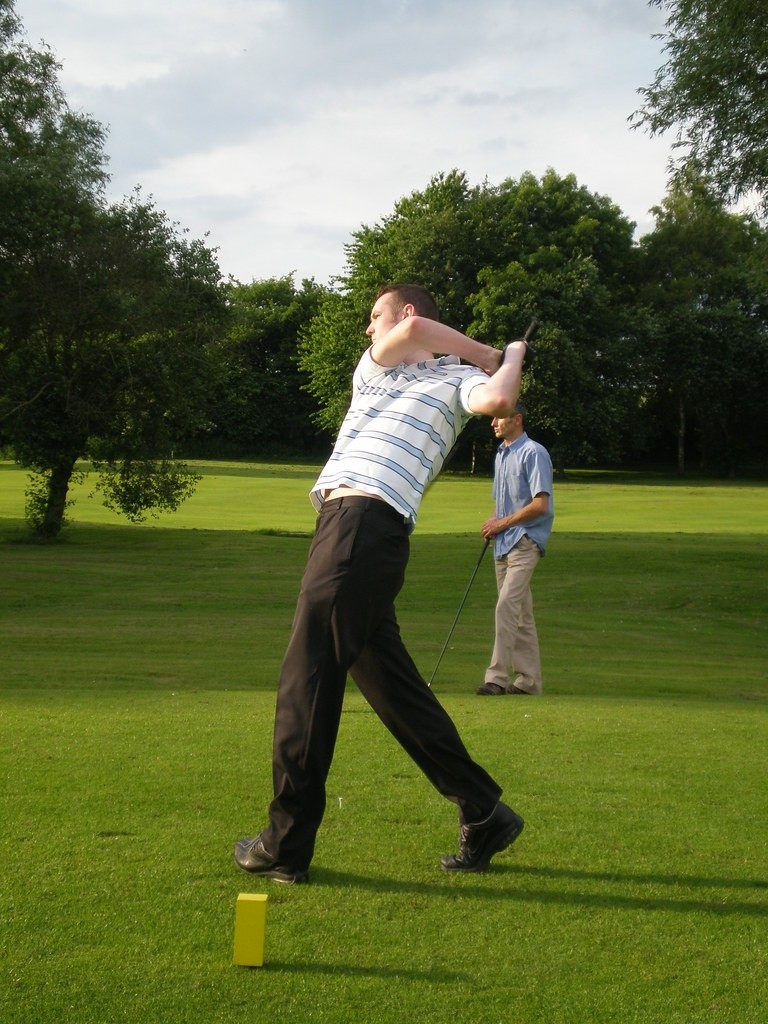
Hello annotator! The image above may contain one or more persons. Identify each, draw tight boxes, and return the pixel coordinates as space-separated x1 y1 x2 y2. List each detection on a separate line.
234 287 525 885
477 406 555 697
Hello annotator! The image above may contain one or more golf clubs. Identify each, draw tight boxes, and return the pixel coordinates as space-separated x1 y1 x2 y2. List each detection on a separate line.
426 537 491 688
523 319 542 344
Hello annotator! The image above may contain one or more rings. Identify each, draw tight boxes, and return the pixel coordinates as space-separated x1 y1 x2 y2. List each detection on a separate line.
484 529 487 533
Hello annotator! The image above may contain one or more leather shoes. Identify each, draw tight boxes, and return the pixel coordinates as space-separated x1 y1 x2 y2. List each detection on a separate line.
234 832 306 883
439 801 524 872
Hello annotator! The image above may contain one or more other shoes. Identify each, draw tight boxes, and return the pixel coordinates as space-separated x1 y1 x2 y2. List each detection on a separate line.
507 683 528 695
476 682 507 696
